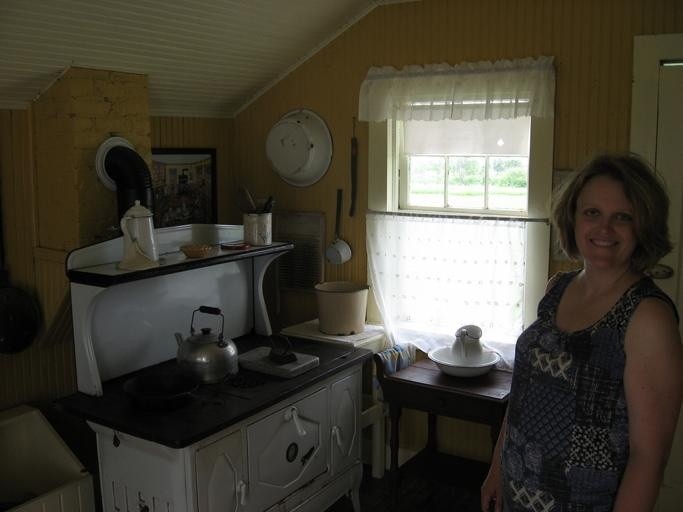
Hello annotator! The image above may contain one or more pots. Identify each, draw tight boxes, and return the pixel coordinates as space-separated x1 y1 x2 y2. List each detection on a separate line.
122 372 225 407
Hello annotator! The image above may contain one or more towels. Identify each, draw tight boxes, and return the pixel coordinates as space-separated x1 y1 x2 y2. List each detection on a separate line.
374 343 416 403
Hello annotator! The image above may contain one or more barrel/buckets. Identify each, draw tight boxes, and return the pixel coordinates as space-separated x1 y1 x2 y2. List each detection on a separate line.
315 278 371 336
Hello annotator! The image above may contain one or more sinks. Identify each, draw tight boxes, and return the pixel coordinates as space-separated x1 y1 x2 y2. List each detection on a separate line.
0 404 95 512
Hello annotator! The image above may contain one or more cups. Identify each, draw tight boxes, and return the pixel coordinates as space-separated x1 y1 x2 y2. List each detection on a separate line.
242 212 272 246
121 199 159 267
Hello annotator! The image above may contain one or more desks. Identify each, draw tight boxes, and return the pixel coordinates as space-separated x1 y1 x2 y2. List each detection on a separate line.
384 358 513 512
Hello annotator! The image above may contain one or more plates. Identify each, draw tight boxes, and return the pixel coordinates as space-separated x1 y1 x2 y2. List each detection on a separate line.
181 245 212 258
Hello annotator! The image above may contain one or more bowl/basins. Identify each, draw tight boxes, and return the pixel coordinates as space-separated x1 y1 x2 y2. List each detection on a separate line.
266 108 333 187
428 346 500 378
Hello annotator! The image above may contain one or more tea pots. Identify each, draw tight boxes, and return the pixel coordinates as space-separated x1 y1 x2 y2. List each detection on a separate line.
174 305 239 385
450 324 484 362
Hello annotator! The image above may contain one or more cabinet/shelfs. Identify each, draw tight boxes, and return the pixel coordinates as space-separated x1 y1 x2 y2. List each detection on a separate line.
193 361 366 512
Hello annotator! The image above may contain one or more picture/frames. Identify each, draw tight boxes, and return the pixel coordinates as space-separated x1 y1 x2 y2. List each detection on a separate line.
151 147 217 228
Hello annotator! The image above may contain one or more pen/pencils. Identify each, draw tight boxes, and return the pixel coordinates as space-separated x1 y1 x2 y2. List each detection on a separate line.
241 185 276 213
